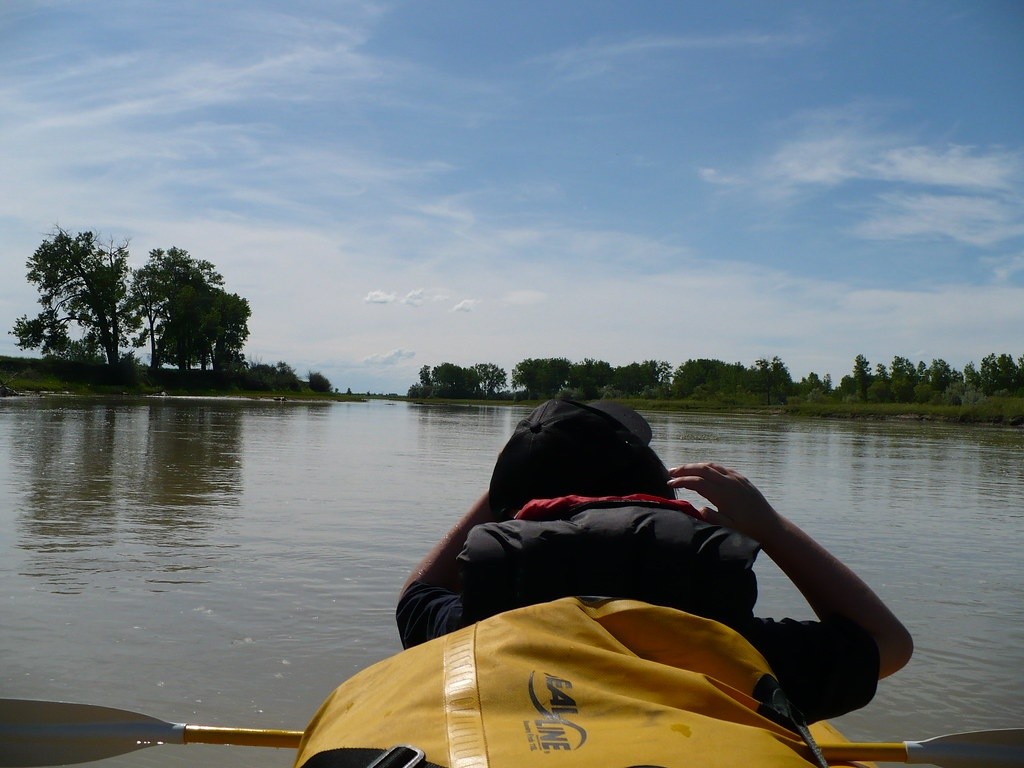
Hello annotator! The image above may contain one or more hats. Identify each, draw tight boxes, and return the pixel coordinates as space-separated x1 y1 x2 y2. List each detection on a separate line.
488 398 652 522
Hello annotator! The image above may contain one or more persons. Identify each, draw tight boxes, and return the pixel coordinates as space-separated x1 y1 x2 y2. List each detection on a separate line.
393 397 915 726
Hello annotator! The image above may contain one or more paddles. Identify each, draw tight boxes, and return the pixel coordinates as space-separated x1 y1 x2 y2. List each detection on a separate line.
0 699 1024 768
